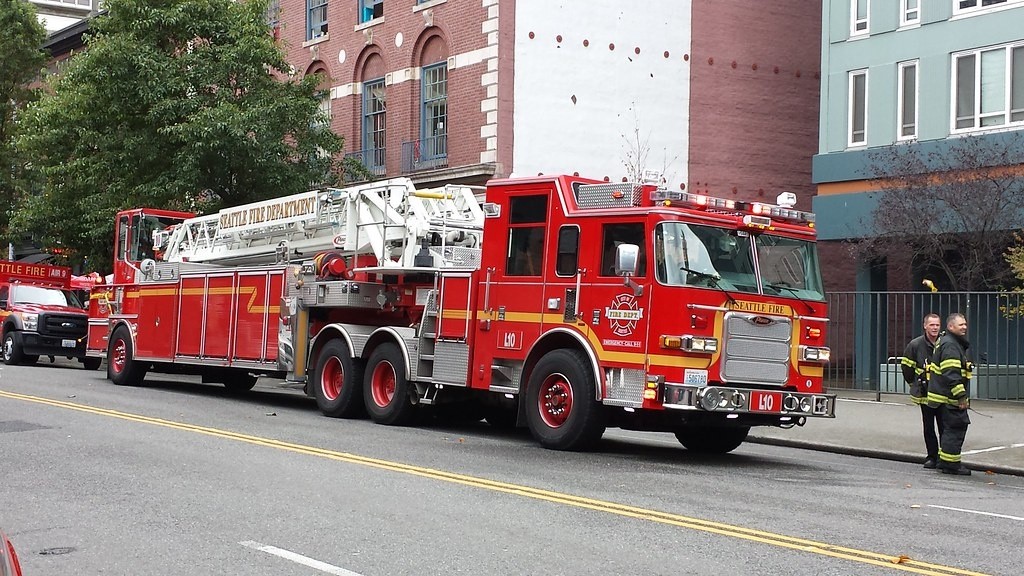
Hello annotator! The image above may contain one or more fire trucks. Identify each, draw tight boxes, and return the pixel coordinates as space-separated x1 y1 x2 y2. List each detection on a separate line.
84 172 839 453
0 260 103 371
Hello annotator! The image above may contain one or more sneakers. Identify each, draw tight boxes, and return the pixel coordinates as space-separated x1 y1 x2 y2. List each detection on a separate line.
942 467 971 475
924 458 939 467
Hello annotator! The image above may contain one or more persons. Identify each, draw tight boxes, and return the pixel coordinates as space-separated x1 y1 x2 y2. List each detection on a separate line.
901 313 975 475
